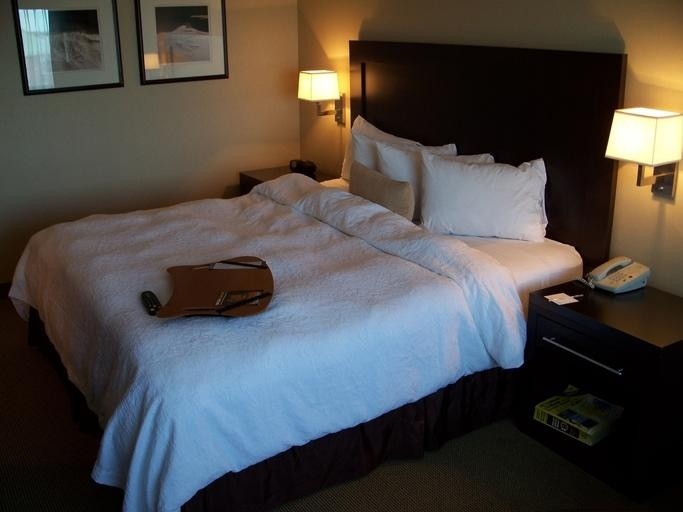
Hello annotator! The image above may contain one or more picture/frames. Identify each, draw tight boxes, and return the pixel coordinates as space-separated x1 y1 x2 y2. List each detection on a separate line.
8 0 123 100
132 1 230 85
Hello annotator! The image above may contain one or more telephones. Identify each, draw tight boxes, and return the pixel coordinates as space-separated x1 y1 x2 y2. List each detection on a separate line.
587 257 650 293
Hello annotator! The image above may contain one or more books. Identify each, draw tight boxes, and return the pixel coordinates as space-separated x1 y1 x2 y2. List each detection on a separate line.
533 385 623 448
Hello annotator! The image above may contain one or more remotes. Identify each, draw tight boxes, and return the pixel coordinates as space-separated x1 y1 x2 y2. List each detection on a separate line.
141 291 161 316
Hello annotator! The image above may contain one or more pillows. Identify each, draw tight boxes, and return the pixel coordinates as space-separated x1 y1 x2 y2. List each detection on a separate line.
348 158 414 222
347 128 457 175
417 149 550 243
374 140 496 227
340 115 457 183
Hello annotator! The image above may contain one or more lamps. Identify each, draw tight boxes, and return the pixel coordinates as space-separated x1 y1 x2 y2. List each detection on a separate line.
296 70 345 128
604 105 683 202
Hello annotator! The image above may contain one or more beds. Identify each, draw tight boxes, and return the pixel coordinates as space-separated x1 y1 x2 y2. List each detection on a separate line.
9 37 629 511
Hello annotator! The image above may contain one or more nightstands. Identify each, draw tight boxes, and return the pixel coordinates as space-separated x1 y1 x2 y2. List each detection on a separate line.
515 273 681 512
237 166 339 194
